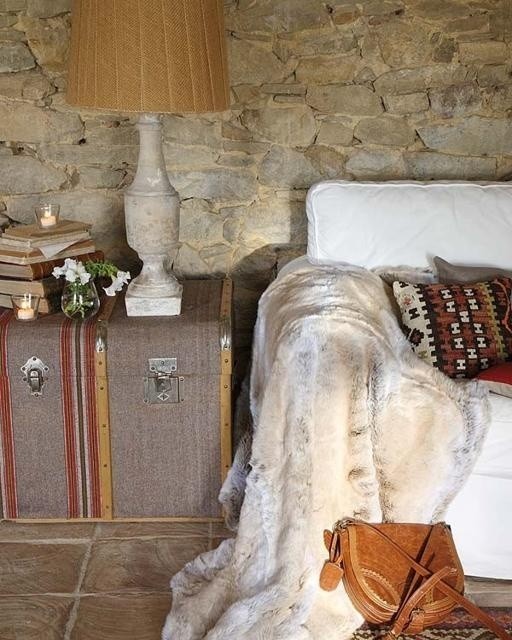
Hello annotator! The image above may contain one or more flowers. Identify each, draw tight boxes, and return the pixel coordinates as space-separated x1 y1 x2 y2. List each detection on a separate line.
52 255 133 316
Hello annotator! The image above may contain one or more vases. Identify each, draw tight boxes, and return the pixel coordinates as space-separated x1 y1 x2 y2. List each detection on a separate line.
60 281 101 320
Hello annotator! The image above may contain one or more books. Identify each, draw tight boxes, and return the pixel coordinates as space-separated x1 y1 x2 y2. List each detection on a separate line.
0 219 104 316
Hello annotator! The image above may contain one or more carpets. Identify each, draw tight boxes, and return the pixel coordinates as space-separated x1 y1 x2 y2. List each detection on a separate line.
347 605 512 639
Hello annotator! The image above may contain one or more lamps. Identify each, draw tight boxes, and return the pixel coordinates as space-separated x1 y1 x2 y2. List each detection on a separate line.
64 0 233 319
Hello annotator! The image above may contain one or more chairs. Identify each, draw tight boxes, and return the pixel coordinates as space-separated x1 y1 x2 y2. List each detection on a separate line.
268 175 510 586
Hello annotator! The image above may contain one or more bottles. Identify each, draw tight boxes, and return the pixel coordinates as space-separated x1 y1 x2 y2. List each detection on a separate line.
61 275 101 322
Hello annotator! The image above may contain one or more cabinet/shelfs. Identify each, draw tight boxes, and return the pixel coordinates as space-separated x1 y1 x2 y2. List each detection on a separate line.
0 273 235 524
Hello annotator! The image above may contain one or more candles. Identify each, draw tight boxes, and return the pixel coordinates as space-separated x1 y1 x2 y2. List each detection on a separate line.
17 308 34 321
40 216 57 226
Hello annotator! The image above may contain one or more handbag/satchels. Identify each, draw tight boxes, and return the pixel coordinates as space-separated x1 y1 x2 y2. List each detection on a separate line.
317 515 511 640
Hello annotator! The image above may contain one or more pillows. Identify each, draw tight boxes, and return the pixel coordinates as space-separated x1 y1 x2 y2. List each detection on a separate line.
376 253 509 399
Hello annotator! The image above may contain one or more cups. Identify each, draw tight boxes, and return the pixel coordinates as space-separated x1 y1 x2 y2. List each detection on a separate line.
11 293 42 323
32 203 61 230
